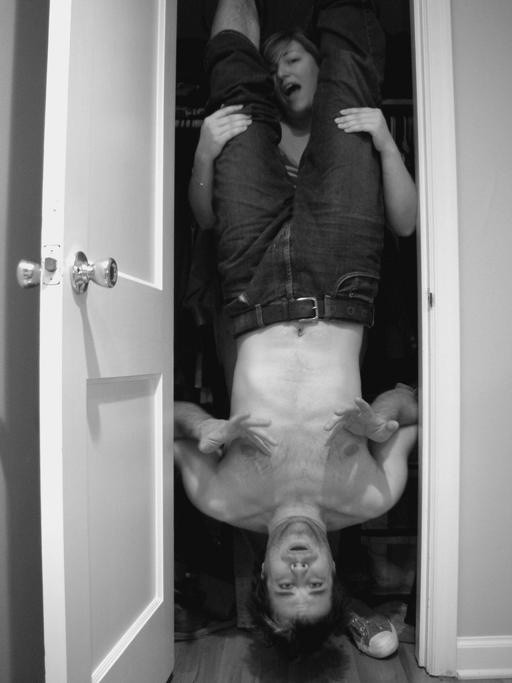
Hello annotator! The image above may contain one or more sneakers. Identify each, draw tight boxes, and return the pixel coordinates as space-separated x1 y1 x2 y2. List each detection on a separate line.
341 599 399 659
174 606 236 639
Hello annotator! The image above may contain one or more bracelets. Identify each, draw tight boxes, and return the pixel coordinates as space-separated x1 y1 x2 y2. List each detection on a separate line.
192 167 214 189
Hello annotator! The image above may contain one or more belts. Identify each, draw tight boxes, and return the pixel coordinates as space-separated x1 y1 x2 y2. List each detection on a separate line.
232 298 373 337
